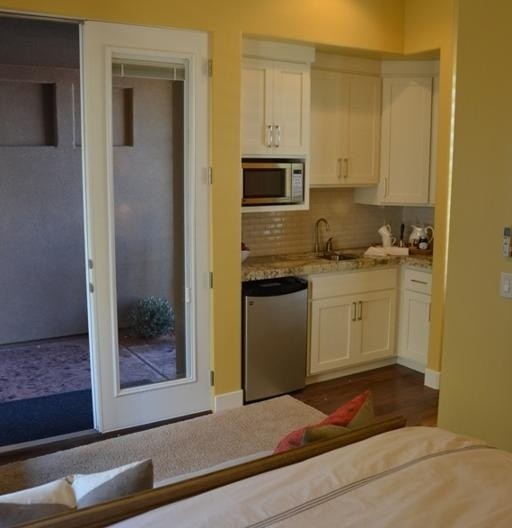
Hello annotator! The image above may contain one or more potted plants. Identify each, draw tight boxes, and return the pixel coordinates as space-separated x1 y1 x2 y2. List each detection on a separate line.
125 295 175 346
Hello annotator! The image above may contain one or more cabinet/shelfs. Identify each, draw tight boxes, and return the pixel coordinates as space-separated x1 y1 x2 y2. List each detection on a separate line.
381 74 434 204
308 62 382 187
309 270 397 374
399 270 430 373
241 56 311 155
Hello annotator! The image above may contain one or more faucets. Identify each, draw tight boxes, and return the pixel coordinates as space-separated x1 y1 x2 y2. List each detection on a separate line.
314 218 329 254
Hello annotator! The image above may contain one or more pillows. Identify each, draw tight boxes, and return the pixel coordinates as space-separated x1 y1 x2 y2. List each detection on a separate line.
73 458 156 508
301 423 349 441
0 479 77 528
273 391 369 452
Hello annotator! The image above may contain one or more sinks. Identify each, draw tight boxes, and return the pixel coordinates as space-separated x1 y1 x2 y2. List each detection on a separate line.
318 253 355 262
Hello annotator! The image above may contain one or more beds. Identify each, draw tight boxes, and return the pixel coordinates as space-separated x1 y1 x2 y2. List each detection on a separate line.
107 425 511 527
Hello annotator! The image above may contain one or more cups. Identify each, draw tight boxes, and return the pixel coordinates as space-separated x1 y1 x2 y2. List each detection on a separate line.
378 225 392 237
382 237 396 248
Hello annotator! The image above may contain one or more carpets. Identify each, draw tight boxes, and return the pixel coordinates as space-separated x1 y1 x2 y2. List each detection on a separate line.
0 395 328 494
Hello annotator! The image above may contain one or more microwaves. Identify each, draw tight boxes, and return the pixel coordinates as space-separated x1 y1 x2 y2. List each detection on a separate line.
241 154 310 213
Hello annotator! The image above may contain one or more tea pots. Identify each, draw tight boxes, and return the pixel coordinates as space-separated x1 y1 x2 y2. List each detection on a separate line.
408 224 434 246
